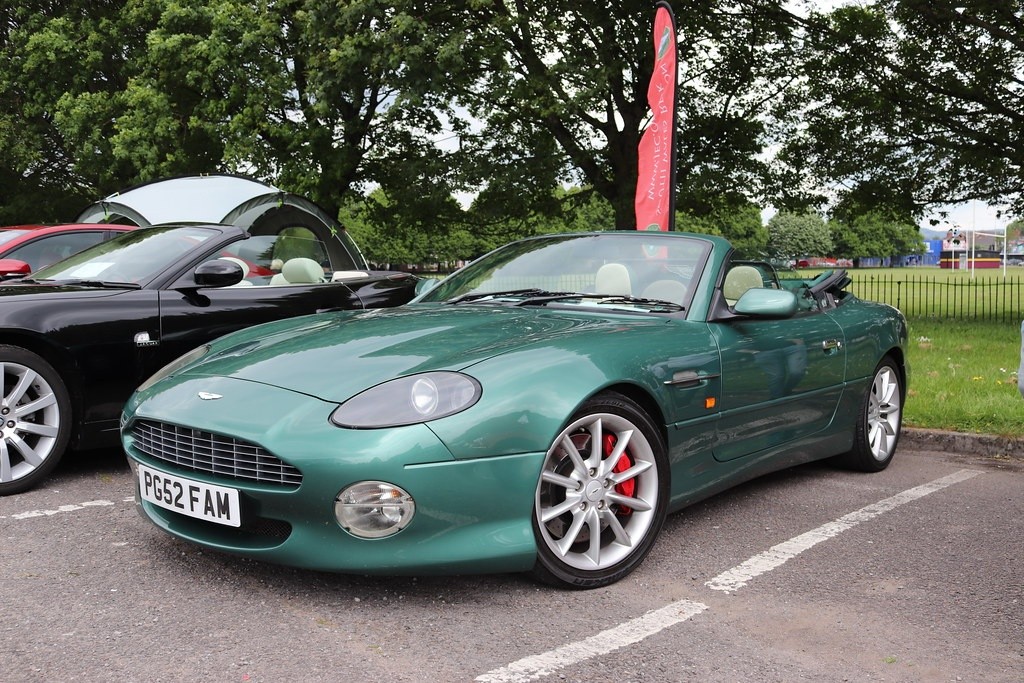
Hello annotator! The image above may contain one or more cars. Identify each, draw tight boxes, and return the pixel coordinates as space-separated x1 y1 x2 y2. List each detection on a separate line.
790 260 810 268
816 260 853 266
1002 258 1024 266
1 224 276 285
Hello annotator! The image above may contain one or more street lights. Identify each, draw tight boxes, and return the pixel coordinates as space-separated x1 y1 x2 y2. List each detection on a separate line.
952 224 959 273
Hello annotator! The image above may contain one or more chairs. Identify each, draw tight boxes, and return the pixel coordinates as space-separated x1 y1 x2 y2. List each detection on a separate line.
213 256 254 286
641 279 687 302
593 262 633 297
720 263 765 309
270 272 289 285
282 255 328 284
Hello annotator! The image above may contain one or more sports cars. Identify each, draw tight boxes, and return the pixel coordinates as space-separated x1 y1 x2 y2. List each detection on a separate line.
119 230 911 590
0 224 427 498
